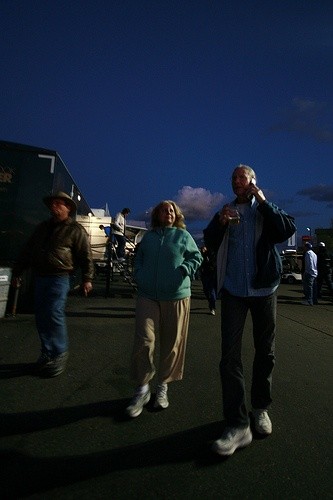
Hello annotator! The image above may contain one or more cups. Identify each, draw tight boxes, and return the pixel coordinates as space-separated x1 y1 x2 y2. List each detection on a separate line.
228 209 240 225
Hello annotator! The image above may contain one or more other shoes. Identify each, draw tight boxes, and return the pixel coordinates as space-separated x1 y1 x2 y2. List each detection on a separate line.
36 352 69 378
211 310 216 316
302 300 312 305
117 257 126 261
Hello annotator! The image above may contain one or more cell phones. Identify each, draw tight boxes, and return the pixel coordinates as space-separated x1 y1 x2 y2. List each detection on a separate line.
247 178 256 200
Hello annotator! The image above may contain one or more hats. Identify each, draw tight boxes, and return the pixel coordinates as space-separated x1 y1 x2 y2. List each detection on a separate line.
317 242 325 247
42 191 78 214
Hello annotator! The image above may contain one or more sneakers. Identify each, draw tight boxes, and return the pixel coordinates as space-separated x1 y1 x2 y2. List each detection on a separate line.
213 426 253 456
155 381 169 408
128 389 151 417
251 408 273 435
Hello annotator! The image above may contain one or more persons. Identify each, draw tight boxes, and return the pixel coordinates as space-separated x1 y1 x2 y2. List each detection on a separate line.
27 192 95 379
199 241 219 316
301 241 327 306
126 200 204 417
111 208 131 262
204 165 296 456
